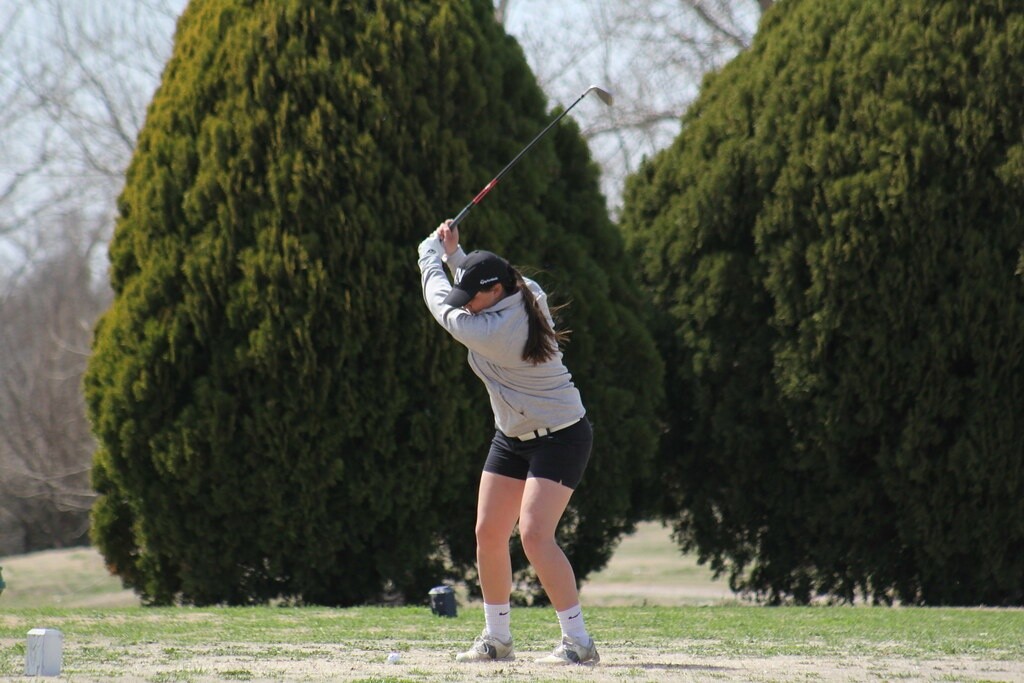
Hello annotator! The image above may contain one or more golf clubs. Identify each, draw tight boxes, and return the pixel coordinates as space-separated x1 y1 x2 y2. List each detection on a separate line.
439 86 614 243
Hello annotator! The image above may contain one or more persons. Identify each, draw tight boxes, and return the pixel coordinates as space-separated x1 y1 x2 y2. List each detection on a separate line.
417 217 602 668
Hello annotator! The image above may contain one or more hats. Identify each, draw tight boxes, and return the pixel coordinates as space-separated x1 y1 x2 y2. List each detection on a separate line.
443 249 511 307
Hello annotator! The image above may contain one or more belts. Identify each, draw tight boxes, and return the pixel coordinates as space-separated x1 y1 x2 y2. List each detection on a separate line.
519 413 584 442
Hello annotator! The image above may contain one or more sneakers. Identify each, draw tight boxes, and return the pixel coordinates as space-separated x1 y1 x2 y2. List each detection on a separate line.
456 632 516 665
534 638 601 671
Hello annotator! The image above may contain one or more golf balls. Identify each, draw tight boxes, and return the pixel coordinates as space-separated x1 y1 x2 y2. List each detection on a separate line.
388 653 399 664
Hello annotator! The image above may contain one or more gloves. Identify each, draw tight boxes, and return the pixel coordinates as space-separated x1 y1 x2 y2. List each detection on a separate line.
418 230 446 260
